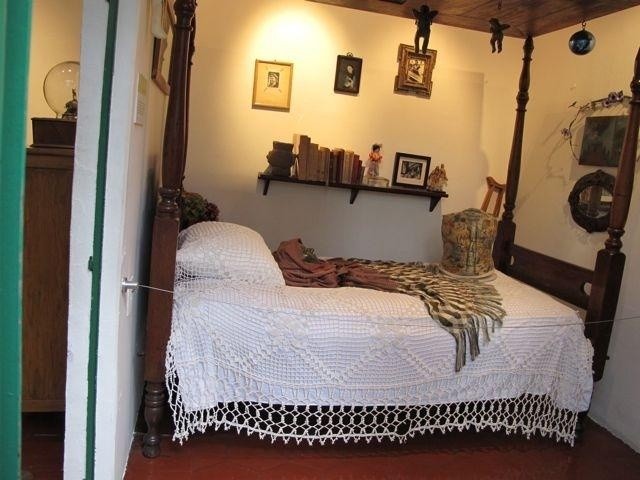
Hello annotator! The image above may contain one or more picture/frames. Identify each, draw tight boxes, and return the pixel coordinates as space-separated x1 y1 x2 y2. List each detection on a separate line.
391 152 431 189
394 43 437 99
151 0 176 96
334 55 362 95
579 115 636 167
252 59 294 112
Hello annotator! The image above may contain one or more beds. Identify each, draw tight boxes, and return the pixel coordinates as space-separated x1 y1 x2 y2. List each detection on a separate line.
144 0 640 458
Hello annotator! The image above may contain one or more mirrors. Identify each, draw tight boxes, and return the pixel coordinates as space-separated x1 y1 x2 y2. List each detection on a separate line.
568 169 625 234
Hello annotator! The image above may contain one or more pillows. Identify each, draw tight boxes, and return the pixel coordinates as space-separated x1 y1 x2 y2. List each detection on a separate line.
176 221 286 287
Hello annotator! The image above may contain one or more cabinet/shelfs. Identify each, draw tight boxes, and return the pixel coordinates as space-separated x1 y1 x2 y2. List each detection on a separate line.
22 148 75 417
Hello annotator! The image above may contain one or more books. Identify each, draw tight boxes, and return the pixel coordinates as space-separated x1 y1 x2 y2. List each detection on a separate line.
293 134 365 185
